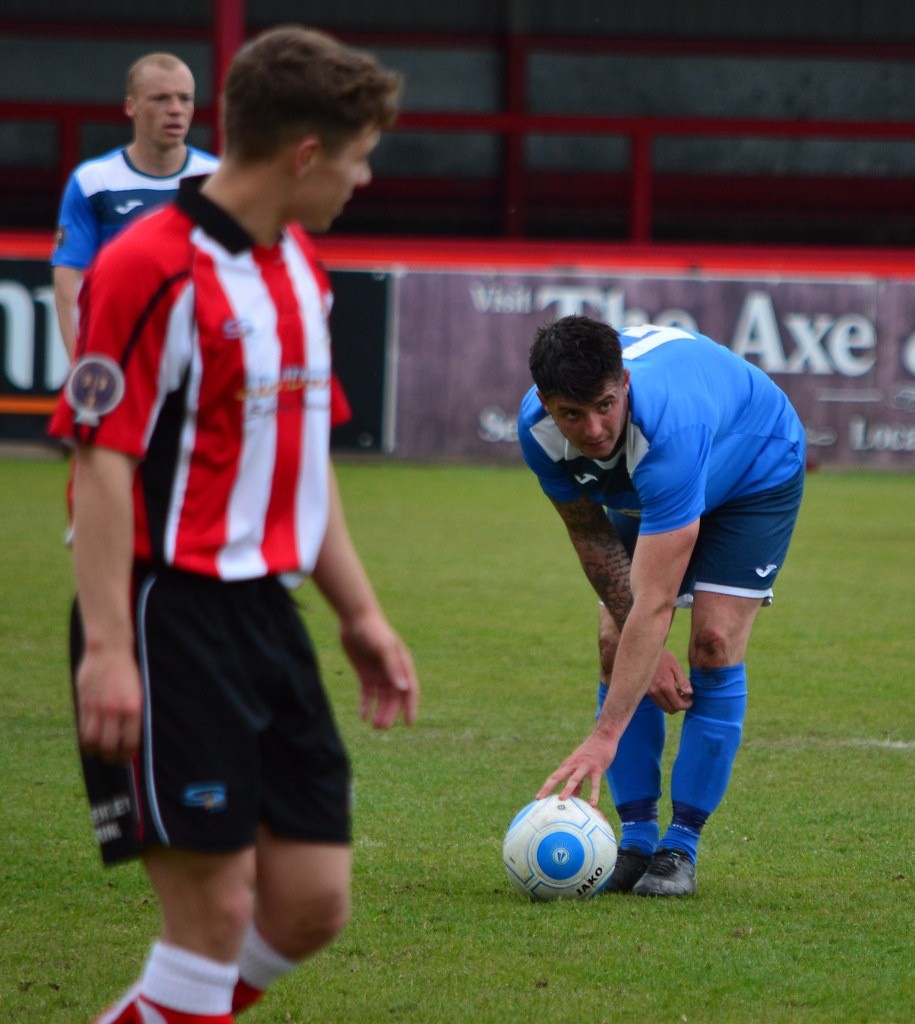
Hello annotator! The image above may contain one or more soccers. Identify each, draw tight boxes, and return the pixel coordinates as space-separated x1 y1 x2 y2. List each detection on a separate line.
502 793 617 903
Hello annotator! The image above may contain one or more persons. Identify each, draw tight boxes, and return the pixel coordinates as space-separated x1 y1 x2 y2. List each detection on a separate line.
48 53 220 363
516 315 807 897
66 27 419 1022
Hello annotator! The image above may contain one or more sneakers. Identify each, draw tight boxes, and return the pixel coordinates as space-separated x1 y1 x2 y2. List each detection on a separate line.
633 853 698 897
597 848 651 894
